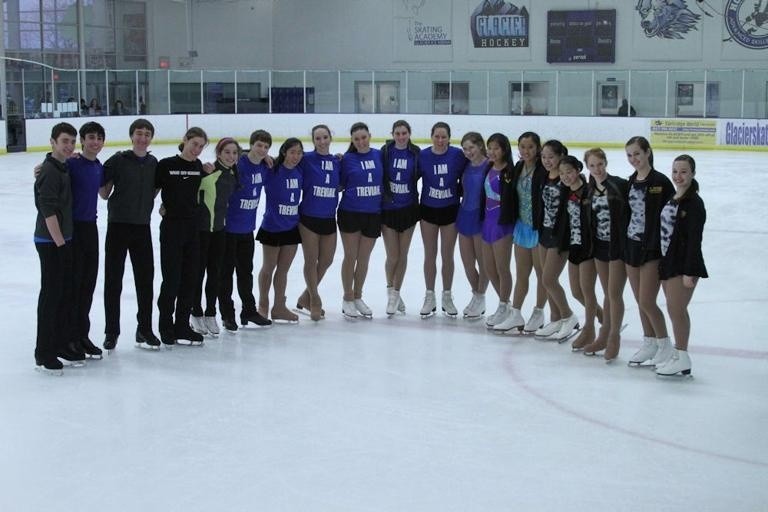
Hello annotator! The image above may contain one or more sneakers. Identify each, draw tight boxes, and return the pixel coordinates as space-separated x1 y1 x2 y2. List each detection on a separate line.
420 289 436 315
80 336 102 355
136 331 160 346
296 295 325 317
584 327 609 353
640 337 672 366
190 314 208 335
555 313 581 340
341 299 358 318
221 317 237 331
103 333 118 350
310 300 322 321
571 326 596 348
486 302 511 326
387 287 405 311
467 294 486 317
493 307 525 331
535 319 561 337
603 332 621 360
655 347 691 375
204 316 220 335
354 299 372 316
161 330 175 345
60 341 85 361
524 307 545 331
629 336 657 363
257 309 269 319
271 307 298 320
441 290 457 315
239 310 272 326
176 326 203 342
463 294 475 315
35 356 63 371
386 290 400 315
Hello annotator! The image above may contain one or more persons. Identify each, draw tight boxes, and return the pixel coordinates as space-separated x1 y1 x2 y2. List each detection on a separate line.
419 122 470 320
626 136 676 367
454 132 493 320
255 138 342 325
655 154 708 376
480 133 513 329
33 119 85 373
492 132 547 335
88 99 105 115
96 107 102 114
39 92 51 103
35 119 161 356
81 99 88 115
296 124 340 322
158 137 274 339
618 99 636 117
337 122 384 321
557 157 629 351
583 148 630 364
71 118 161 356
535 140 583 344
112 100 131 115
381 120 420 320
204 129 273 334
154 127 207 350
67 97 75 102
